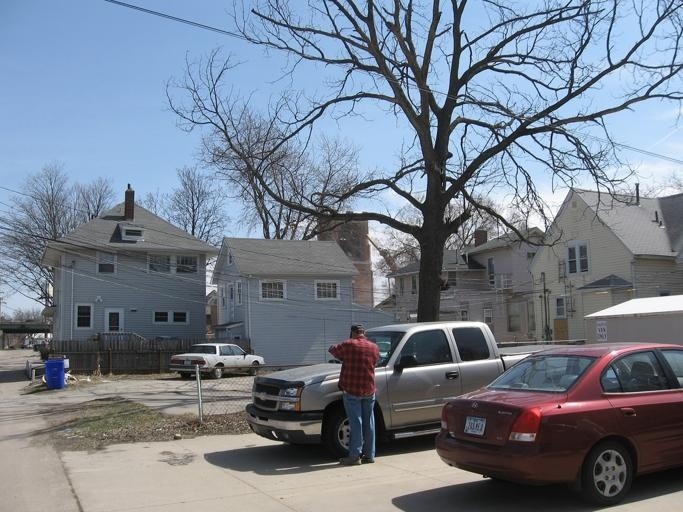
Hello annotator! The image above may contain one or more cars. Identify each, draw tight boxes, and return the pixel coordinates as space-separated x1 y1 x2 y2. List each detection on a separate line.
168 340 266 379
436 344 682 506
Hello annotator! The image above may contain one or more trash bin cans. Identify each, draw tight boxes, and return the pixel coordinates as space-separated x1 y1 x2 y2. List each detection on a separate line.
44 359 65 390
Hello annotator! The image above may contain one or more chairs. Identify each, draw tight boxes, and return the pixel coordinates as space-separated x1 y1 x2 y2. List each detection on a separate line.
630 362 656 389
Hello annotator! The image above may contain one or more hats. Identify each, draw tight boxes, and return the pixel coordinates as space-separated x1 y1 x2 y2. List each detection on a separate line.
351 324 363 331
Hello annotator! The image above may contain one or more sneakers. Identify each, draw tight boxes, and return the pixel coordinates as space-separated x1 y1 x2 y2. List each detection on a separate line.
361 455 374 464
339 457 361 464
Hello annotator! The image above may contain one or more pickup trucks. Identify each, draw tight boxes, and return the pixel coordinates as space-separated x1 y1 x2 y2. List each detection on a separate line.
246 316 581 454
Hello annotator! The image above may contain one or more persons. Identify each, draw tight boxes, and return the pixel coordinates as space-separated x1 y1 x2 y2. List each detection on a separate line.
328 323 379 466
